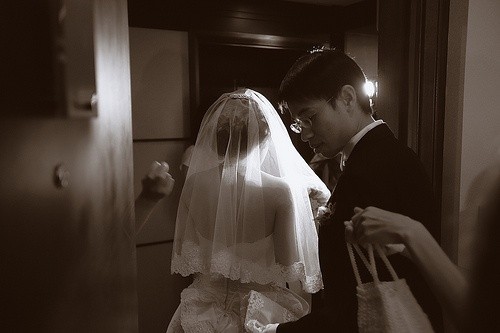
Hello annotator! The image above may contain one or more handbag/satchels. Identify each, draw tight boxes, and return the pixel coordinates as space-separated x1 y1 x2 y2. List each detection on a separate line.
345 219 435 333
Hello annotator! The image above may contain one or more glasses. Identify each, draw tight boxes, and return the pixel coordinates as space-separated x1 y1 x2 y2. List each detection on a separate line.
290 94 337 134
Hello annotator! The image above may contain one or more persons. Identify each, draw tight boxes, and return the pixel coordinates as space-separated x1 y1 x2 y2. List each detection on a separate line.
170 90 324 333
259 50 433 333
345 206 473 333
